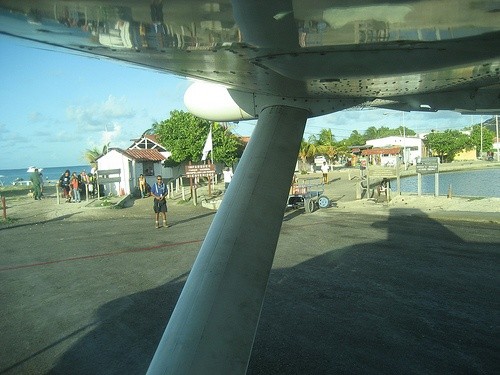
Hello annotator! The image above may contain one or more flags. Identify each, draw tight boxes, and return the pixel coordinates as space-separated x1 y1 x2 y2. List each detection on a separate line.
201 130 212 161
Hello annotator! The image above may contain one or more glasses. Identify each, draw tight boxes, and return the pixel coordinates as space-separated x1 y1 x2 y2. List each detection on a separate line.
158 179 162 180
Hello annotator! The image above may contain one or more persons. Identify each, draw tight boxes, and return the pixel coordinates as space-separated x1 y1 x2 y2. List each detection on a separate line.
321 162 329 182
30 169 44 200
151 175 168 228
58 170 96 203
377 178 390 197
222 167 233 189
138 174 146 198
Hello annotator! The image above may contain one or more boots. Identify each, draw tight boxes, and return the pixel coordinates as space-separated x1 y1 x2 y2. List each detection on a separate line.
155 223 159 228
163 222 169 228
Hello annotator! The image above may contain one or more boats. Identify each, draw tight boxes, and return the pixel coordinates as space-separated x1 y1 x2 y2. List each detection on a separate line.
27 166 44 173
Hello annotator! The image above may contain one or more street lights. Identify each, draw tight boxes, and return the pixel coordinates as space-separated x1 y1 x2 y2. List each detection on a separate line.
383 111 407 171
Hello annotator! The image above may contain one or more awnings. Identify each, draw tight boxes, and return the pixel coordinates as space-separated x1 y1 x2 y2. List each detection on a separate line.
347 145 373 155
361 145 402 156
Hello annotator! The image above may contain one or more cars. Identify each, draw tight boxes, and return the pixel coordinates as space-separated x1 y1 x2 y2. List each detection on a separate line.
314 156 327 165
